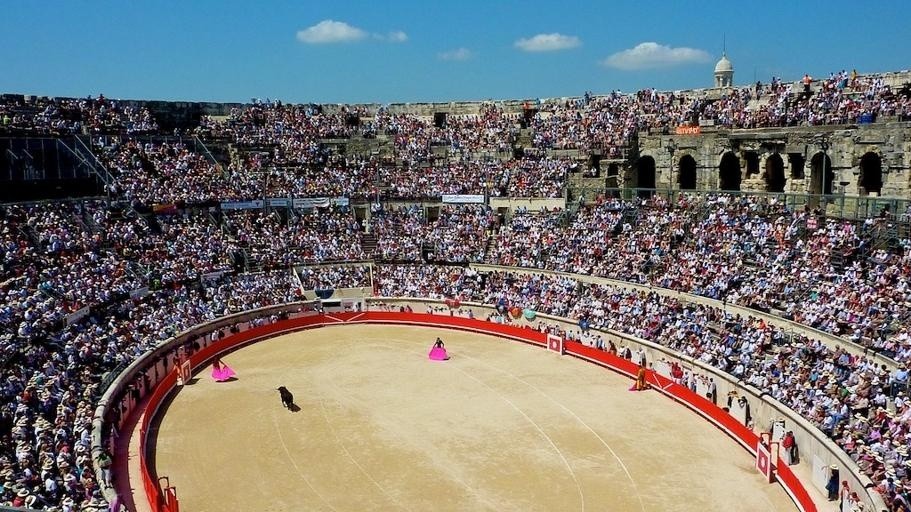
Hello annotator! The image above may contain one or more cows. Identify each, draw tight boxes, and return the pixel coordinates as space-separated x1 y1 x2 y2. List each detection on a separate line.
274 384 293 411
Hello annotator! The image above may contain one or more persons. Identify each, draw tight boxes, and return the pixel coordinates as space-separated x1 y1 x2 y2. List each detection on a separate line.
2 69 911 512
